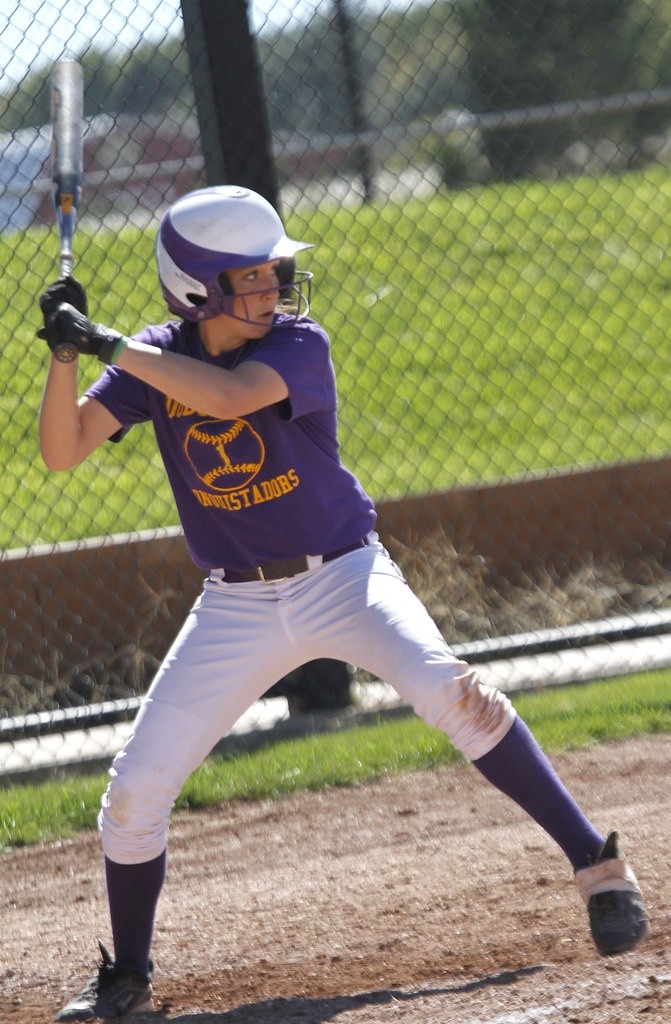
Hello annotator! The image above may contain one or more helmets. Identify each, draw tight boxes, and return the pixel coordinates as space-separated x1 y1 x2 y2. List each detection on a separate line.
155 186 316 320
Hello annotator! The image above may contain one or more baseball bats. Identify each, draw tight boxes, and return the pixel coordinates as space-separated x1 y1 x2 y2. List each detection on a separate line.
51 58 86 364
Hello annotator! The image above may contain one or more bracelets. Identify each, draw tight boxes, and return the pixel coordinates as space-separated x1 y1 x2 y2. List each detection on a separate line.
110 336 128 365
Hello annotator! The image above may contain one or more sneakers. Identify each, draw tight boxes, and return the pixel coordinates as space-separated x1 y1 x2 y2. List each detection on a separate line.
573 829 649 956
52 938 153 1024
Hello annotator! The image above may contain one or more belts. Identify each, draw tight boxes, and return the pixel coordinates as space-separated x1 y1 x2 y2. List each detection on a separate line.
221 536 369 583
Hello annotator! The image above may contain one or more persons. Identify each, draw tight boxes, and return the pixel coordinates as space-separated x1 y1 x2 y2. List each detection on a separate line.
35 186 648 1024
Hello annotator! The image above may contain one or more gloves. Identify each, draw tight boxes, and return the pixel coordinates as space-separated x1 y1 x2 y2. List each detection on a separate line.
39 274 95 356
34 301 123 365
